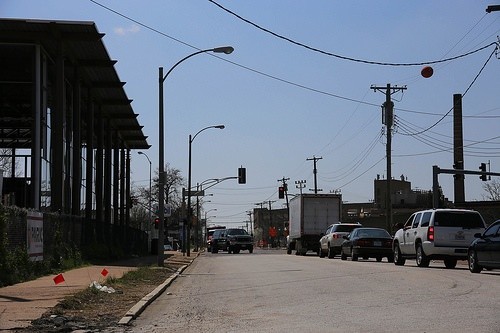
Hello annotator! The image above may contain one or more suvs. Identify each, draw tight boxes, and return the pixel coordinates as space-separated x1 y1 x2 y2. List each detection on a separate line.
317 221 365 259
206 227 254 254
391 208 488 269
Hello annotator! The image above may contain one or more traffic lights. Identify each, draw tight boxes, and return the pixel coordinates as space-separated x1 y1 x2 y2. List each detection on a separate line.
479 163 487 181
278 187 285 199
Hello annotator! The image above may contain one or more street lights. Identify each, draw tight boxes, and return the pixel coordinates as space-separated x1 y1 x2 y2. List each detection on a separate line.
195 182 217 252
157 46 235 267
137 151 152 257
187 125 226 256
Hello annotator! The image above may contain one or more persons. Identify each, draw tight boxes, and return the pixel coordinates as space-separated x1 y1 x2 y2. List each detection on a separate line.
269 225 278 247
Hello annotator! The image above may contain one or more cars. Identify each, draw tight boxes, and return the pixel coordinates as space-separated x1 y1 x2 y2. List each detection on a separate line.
340 227 395 263
467 219 500 274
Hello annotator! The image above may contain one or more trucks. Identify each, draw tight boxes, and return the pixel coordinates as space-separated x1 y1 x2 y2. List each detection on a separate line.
285 193 343 256
